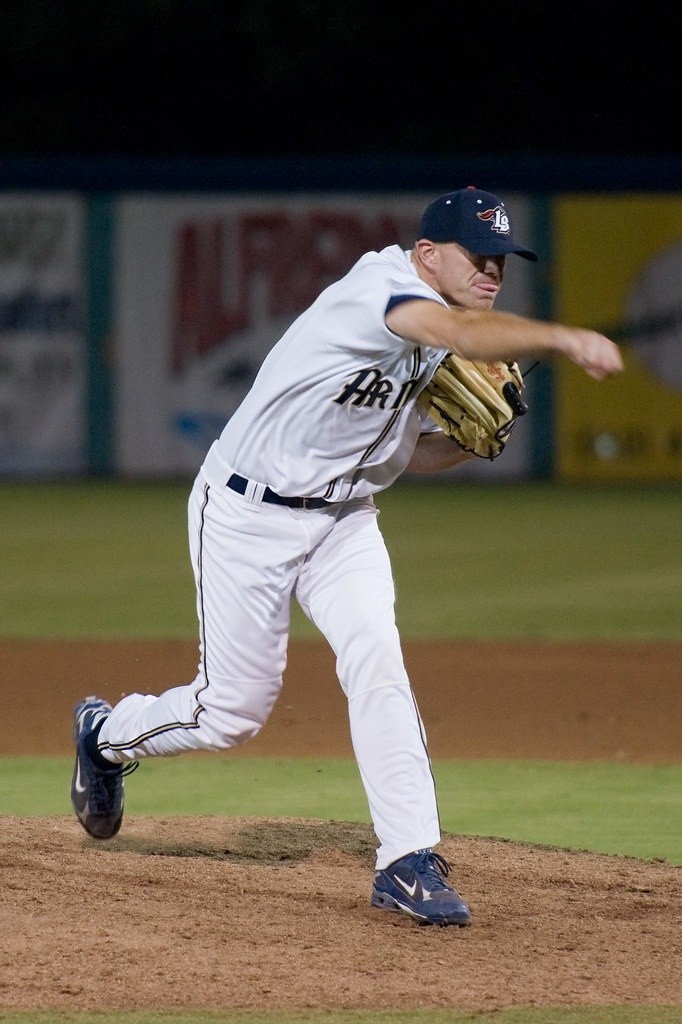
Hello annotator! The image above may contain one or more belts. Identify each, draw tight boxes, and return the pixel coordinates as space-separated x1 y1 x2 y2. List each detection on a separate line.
224 473 338 509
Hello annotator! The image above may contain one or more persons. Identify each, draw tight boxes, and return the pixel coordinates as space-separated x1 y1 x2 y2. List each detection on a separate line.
71 185 624 927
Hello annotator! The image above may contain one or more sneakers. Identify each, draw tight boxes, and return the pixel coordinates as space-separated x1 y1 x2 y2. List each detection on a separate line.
369 848 472 928
69 693 139 841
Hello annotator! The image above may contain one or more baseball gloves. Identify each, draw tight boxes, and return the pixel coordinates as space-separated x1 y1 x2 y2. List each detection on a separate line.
416 350 529 460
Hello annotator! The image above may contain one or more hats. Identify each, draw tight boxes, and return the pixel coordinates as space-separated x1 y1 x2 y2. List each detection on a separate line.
416 184 541 264
